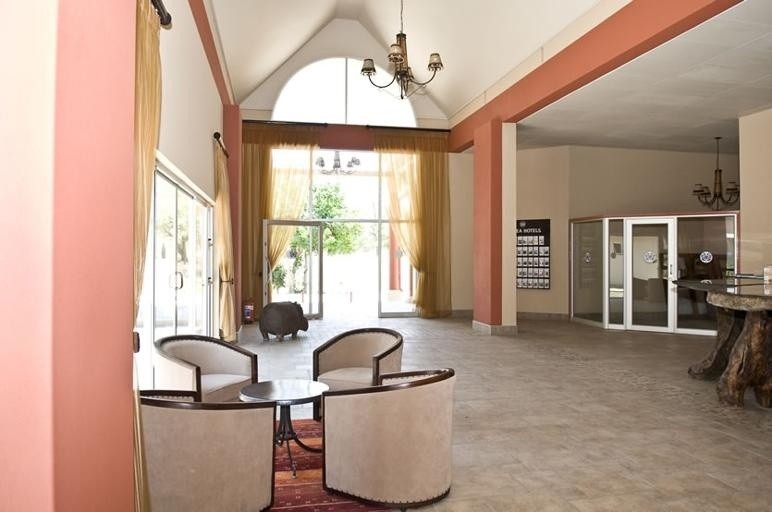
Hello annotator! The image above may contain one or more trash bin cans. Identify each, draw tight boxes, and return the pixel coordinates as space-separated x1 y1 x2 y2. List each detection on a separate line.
243 299 255 324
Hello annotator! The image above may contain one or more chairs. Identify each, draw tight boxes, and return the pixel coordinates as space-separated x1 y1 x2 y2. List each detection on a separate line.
322 368 457 509
141 390 278 510
146 334 259 403
312 328 405 424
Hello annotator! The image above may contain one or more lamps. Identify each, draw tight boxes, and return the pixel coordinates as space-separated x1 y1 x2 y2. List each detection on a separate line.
690 135 740 210
359 1 444 102
316 151 361 177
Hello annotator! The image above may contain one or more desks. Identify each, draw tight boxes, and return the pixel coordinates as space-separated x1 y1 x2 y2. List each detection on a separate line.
239 378 331 478
669 274 771 411
